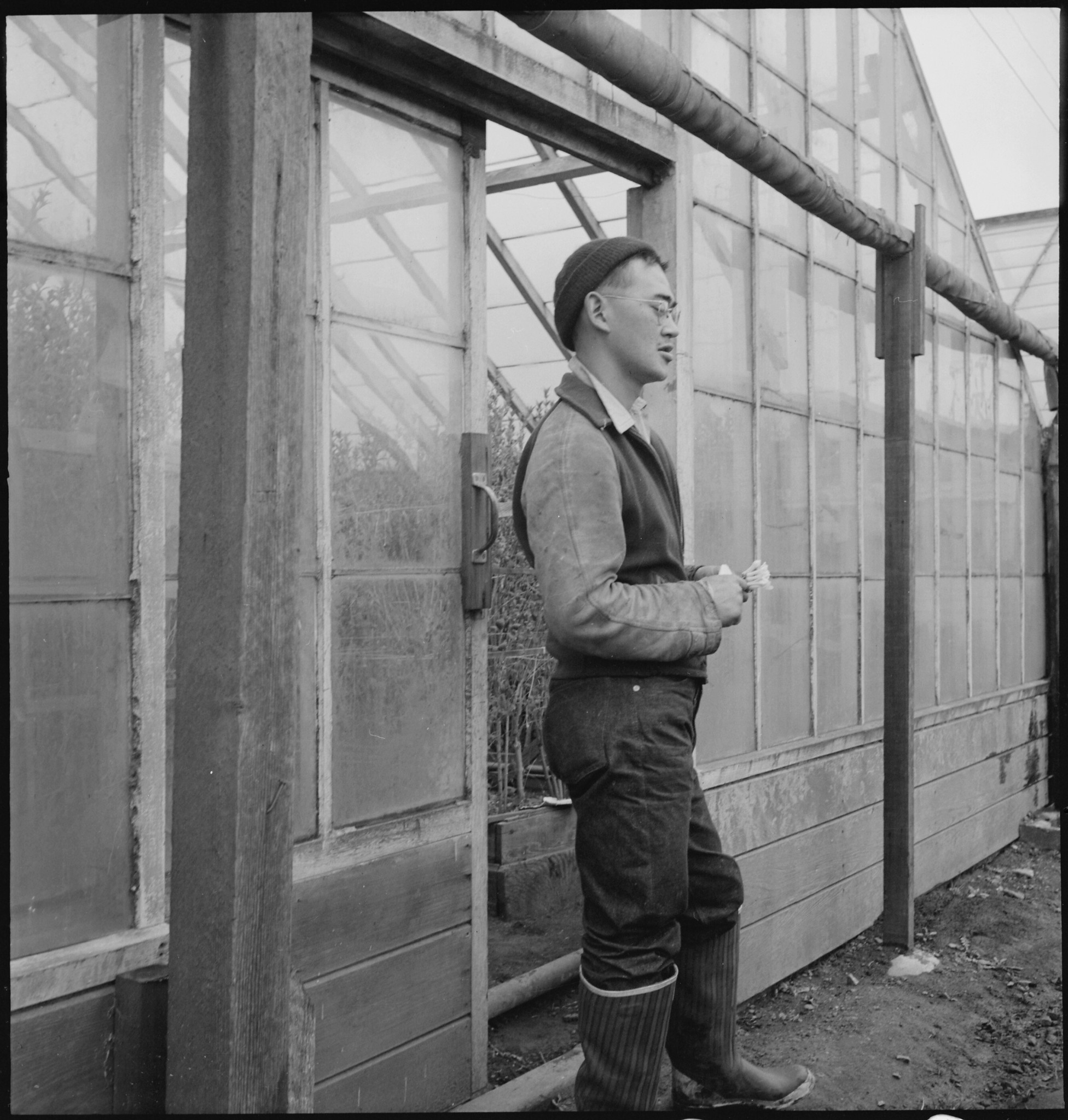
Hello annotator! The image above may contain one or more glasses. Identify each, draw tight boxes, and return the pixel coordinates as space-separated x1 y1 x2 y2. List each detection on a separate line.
603 294 680 326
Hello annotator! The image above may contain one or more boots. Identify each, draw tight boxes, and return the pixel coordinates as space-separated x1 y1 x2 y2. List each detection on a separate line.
670 910 817 1114
572 959 678 1112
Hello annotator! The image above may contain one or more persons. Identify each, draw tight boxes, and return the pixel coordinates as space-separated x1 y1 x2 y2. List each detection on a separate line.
512 235 814 1114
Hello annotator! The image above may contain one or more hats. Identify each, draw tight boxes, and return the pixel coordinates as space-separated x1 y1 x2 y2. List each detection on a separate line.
557 236 659 353
